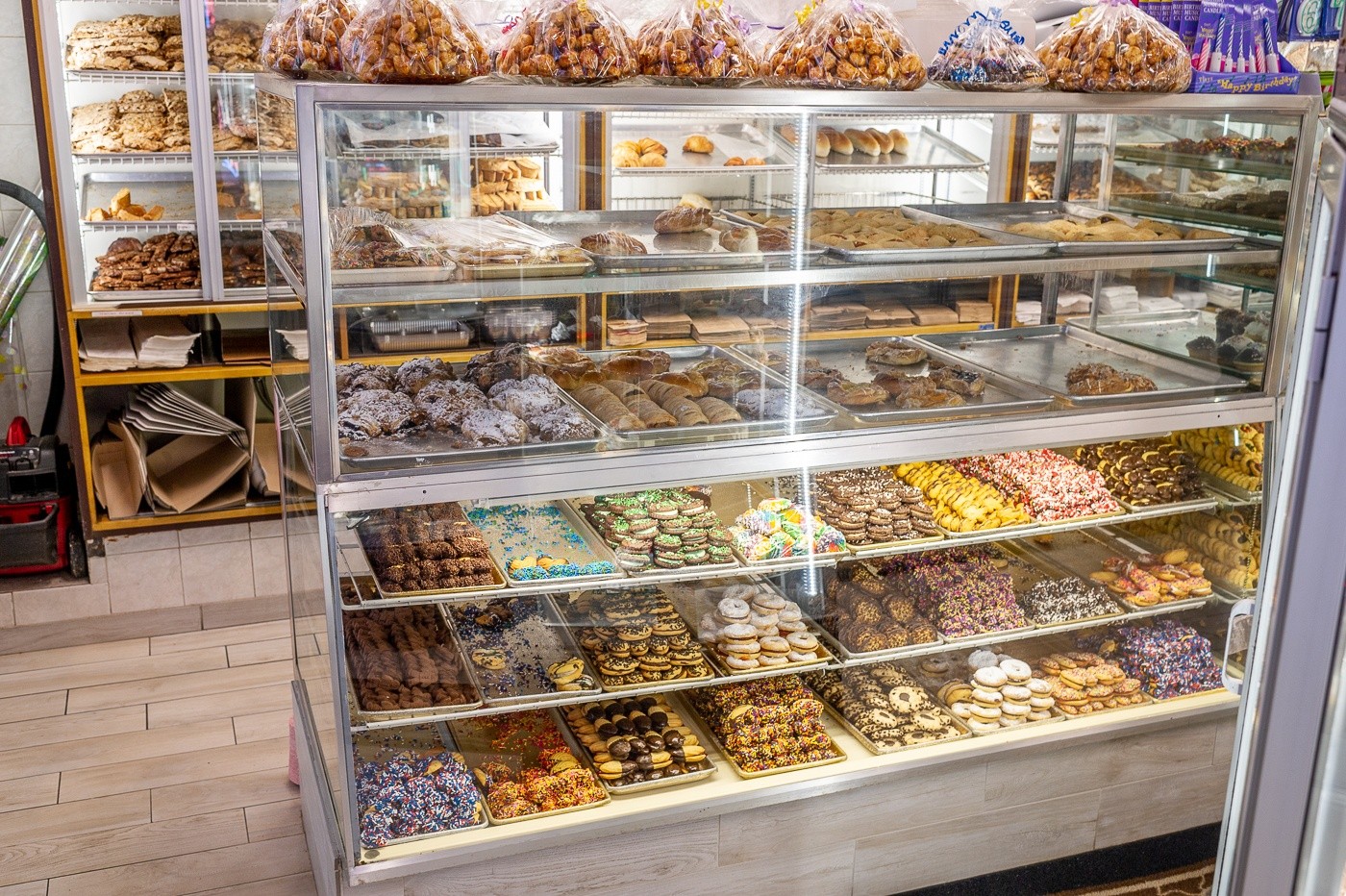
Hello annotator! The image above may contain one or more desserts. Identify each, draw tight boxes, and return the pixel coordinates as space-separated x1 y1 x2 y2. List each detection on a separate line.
368 426 1267 711
354 746 606 848
1158 134 1300 373
564 612 1221 791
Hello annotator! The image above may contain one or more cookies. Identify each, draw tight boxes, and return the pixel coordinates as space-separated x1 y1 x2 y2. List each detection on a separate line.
338 580 470 709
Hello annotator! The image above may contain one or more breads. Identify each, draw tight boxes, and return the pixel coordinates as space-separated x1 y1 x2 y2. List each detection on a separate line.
86 176 262 220
331 114 1233 450
263 0 753 82
759 4 1190 96
91 230 302 290
65 16 297 154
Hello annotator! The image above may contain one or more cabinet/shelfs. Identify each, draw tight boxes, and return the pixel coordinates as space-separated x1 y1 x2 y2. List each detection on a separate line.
21 4 1327 896
